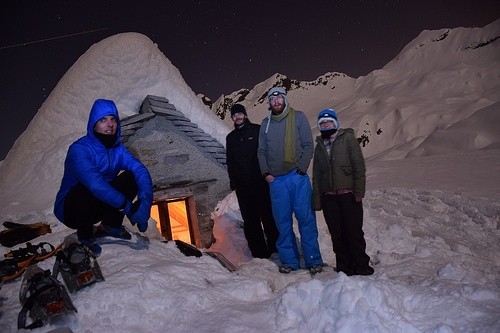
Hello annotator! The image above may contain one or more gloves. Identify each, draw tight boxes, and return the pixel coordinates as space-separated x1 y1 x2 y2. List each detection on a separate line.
124 200 152 231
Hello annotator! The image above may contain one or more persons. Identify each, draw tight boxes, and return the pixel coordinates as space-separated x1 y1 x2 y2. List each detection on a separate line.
312 108 374 276
53 99 154 253
257 86 323 276
226 103 280 259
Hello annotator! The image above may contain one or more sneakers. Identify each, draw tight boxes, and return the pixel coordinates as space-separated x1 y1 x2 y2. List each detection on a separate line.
78 240 102 257
98 224 132 240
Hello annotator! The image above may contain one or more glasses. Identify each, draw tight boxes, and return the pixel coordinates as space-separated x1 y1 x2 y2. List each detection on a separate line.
270 96 284 102
319 120 333 125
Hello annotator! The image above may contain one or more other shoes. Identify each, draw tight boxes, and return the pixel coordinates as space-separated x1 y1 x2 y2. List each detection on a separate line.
278 265 292 274
310 264 323 274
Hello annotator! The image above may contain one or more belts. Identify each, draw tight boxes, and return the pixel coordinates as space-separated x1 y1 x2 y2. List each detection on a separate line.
324 189 352 195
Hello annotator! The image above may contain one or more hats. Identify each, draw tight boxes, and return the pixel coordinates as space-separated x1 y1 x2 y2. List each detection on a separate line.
265 87 288 134
230 103 247 129
317 109 338 130
